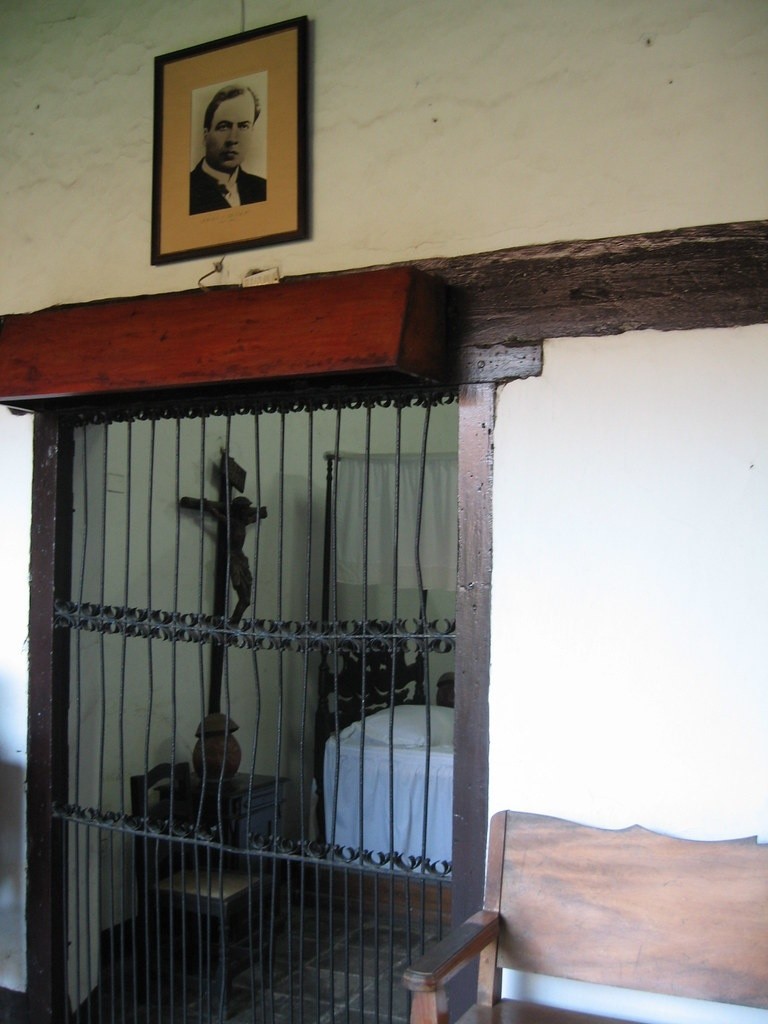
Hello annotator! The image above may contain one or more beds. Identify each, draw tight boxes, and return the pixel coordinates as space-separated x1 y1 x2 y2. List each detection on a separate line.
314 448 458 877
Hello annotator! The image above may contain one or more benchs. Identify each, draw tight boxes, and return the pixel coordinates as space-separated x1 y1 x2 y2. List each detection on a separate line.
401 810 768 1024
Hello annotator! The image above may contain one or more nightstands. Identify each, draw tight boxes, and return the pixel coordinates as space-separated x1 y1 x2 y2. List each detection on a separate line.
152 774 292 944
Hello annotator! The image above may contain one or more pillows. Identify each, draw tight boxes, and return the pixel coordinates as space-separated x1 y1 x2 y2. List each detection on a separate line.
339 703 453 749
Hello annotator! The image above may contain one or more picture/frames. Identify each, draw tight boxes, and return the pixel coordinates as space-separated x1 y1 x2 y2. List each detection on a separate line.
150 13 311 264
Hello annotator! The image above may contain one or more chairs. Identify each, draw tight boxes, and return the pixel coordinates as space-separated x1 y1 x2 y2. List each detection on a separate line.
132 759 278 1003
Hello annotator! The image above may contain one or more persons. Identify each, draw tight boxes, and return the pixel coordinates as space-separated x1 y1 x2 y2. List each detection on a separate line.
199 494 268 645
190 84 267 215
